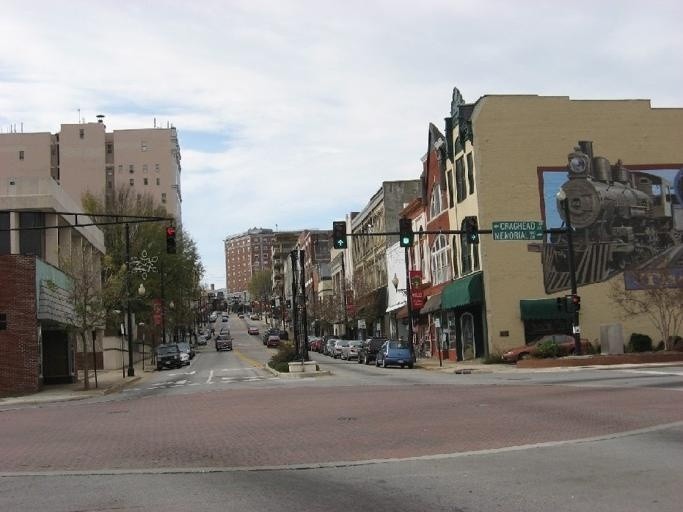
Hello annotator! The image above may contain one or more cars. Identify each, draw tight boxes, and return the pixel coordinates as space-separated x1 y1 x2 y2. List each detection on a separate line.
209 311 219 322
307 334 413 370
214 327 234 351
500 334 591 365
263 327 289 347
248 312 259 320
222 311 229 323
247 325 259 335
237 312 244 319
154 328 212 371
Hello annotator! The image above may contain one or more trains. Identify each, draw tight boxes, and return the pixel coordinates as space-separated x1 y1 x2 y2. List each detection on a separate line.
541 141 682 295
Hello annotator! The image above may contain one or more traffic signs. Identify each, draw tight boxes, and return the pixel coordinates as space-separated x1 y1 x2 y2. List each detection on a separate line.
160 260 175 344
492 221 544 241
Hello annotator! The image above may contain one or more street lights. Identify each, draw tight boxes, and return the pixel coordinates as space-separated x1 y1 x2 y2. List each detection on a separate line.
392 248 414 350
125 223 146 377
556 187 582 357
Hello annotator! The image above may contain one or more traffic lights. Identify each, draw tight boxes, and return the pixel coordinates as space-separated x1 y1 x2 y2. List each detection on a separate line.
557 294 580 313
399 218 414 247
165 226 176 254
333 221 347 250
464 215 479 245
